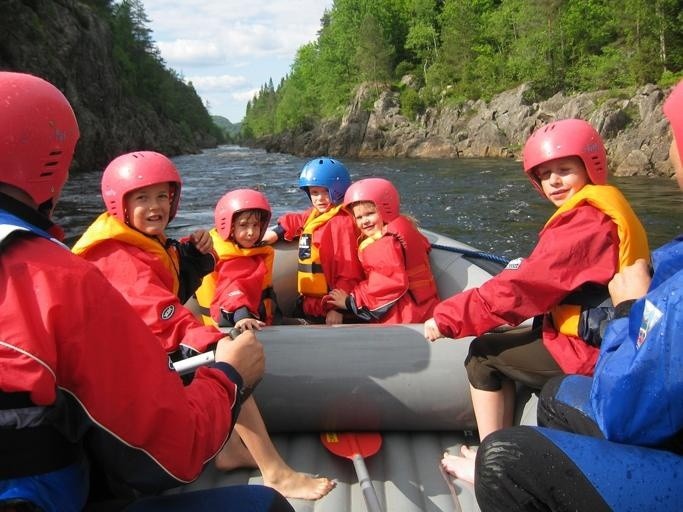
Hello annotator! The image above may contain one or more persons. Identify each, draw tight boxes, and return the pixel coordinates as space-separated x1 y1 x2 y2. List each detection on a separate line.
194 189 284 334
474 78 683 512
326 178 442 325
71 150 336 500
261 155 369 325
423 119 649 484
0 71 295 512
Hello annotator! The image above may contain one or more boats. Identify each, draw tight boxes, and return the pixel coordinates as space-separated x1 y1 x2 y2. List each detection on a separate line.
155 224 538 509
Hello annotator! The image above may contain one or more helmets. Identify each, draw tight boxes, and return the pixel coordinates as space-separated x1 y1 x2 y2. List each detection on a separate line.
663 78 682 164
344 178 400 221
523 120 608 199
1 71 80 204
299 157 352 205
215 189 272 243
102 152 181 226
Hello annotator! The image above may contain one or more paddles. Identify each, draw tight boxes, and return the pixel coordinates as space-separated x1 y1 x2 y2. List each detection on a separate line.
319 432 382 512
